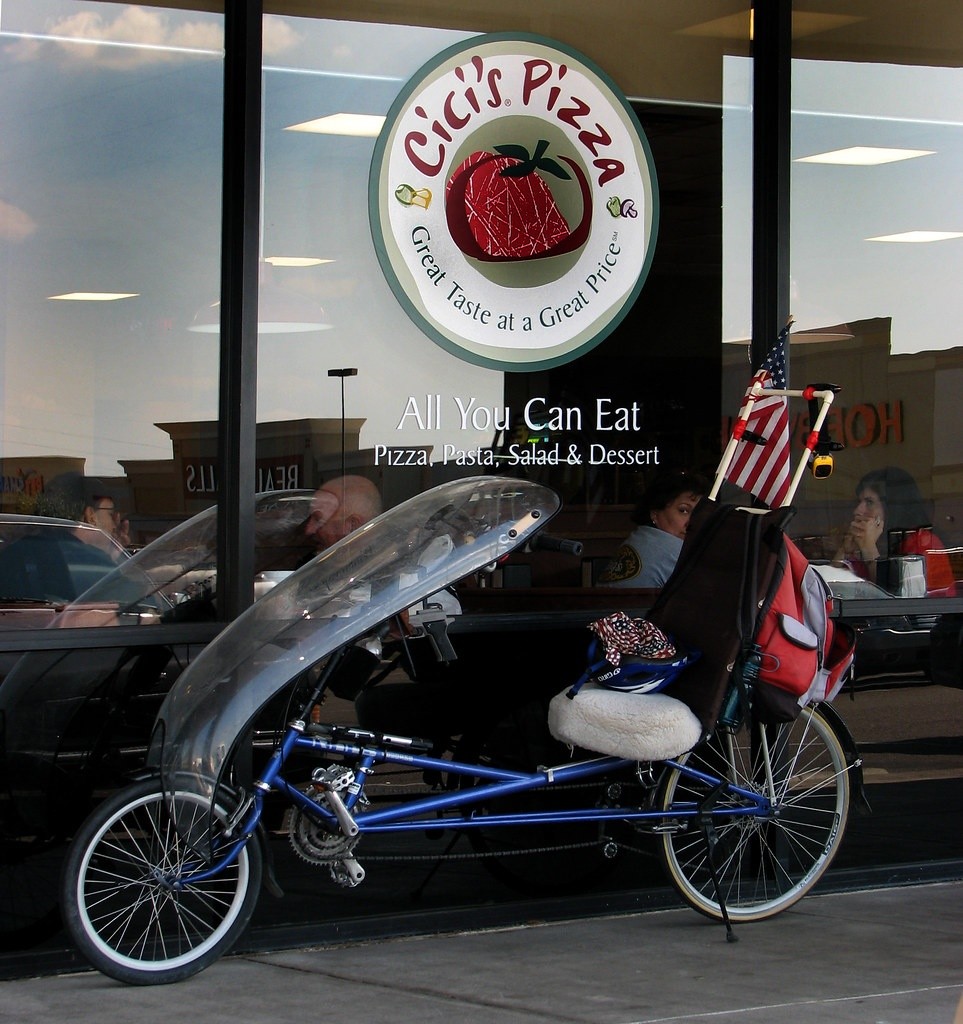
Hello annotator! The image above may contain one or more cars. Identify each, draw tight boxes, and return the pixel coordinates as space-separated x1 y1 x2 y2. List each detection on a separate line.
124 542 370 623
0 509 206 715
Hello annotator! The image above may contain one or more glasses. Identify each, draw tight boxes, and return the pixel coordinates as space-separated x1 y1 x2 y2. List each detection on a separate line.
92 504 119 522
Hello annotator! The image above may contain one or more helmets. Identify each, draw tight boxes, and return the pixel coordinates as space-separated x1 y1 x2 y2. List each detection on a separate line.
587 634 702 695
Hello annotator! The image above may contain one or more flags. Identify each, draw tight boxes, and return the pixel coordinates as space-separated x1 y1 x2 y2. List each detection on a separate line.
715 322 795 509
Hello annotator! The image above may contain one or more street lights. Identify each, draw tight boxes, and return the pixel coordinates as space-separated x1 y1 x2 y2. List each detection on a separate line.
325 367 358 537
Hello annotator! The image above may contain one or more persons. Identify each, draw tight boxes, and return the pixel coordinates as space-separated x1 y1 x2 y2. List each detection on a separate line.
303 474 463 615
831 465 958 597
182 490 219 570
594 479 708 588
0 472 163 614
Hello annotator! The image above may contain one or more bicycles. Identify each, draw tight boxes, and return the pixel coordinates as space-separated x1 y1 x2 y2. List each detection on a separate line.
52 317 869 990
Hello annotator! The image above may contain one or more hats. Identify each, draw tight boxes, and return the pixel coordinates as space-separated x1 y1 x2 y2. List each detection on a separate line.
40 470 128 506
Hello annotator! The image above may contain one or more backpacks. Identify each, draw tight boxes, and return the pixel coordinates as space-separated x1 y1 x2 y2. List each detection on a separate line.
742 531 858 724
